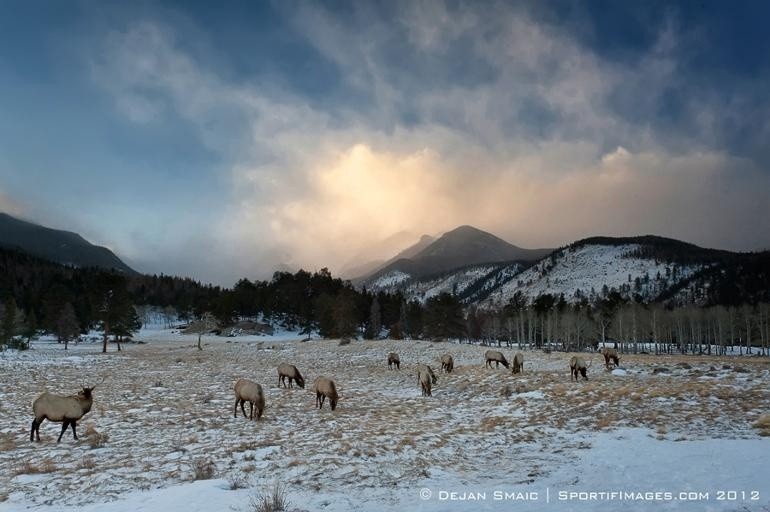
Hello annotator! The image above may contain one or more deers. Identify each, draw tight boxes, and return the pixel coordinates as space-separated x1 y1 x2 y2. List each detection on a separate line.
604 347 621 369
277 362 306 389
569 356 588 381
441 354 453 373
315 376 342 411
485 350 523 375
388 353 400 370
234 378 265 420
30 375 105 442
417 364 438 397
